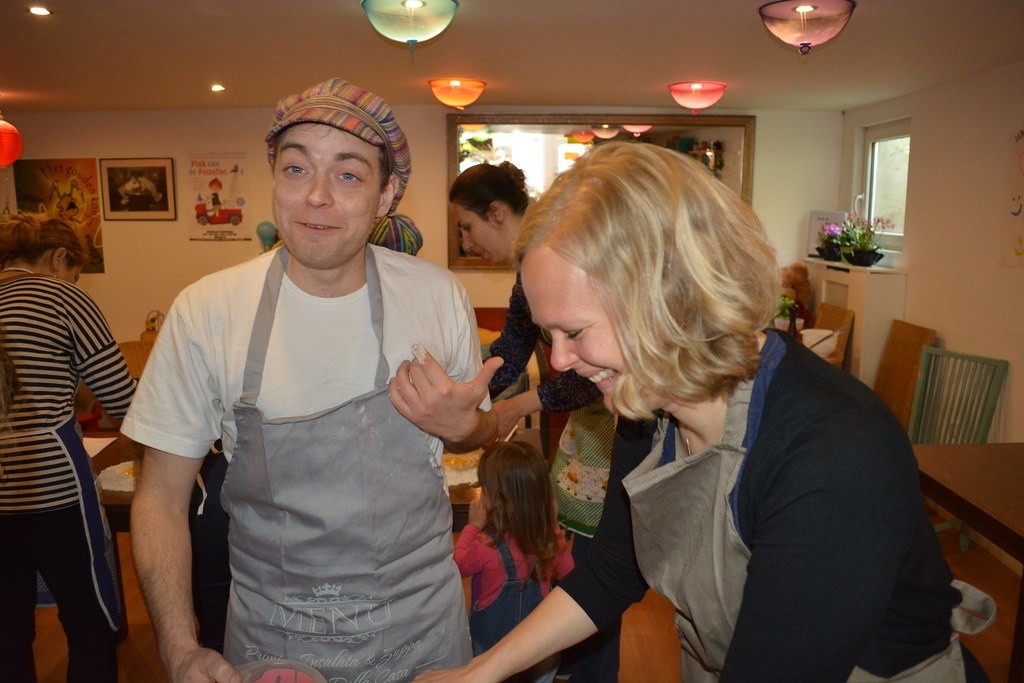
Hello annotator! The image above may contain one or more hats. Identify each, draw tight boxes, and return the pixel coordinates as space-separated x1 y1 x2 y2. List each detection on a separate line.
265 77 412 217
367 214 423 255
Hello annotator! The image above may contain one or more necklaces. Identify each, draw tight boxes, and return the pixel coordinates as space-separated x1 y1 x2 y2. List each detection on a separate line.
684 423 725 456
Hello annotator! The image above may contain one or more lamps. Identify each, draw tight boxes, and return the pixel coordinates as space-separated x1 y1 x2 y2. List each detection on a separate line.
362 0 458 56
572 129 594 145
429 78 486 110
623 125 652 137
591 125 620 139
759 0 856 55
668 81 727 115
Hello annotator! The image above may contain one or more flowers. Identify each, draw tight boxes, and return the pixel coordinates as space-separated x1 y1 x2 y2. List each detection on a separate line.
815 223 843 248
840 211 896 265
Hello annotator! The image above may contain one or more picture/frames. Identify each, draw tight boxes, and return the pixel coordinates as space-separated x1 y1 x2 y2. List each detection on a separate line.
99 157 176 220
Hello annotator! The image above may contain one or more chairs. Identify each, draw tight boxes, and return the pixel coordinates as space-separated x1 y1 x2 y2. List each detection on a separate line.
908 344 1009 445
829 338 833 339
873 319 937 431
815 302 855 371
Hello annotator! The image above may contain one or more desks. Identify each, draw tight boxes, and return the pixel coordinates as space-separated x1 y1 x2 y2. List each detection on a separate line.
81 426 541 532
908 442 1024 683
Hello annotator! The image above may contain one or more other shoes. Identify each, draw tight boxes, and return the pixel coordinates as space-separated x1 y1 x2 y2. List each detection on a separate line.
121 199 128 204
154 193 163 201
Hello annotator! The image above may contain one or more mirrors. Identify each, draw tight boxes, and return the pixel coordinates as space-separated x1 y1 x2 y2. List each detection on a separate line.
446 113 757 272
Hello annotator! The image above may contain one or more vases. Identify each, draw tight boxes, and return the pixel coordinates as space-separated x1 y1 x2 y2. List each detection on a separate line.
843 251 885 266
816 247 841 261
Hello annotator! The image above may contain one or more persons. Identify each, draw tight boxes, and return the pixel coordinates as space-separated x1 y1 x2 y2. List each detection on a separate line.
409 140 999 683
0 212 139 683
119 77 505 683
447 159 659 683
452 441 575 683
